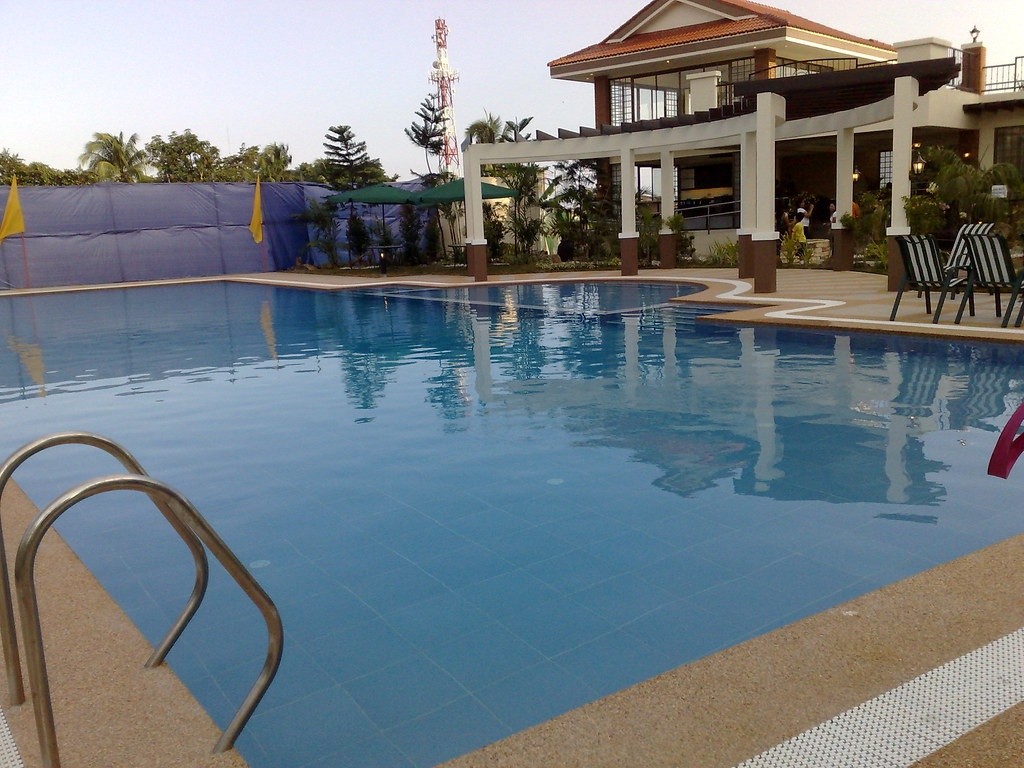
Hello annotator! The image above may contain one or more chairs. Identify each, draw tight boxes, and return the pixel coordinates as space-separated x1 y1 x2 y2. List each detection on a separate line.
889 223 1024 328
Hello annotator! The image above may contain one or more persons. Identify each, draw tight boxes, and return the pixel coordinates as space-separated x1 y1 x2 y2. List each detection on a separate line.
776 210 788 257
794 195 814 240
792 212 807 264
828 200 836 257
852 197 860 221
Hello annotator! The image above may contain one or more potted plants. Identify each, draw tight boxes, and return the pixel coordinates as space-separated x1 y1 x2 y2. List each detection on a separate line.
549 218 582 262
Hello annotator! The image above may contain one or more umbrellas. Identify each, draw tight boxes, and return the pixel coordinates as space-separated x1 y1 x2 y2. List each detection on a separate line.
409 177 520 241
322 182 413 276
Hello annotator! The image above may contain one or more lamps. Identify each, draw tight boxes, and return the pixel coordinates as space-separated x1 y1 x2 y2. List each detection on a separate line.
853 166 861 184
912 151 927 178
969 25 981 43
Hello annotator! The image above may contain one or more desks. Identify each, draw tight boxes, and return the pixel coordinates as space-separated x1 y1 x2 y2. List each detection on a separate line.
448 244 466 269
367 245 402 270
